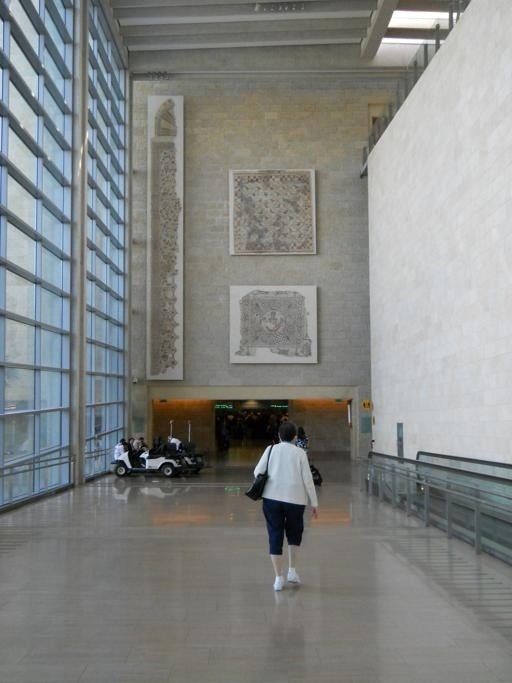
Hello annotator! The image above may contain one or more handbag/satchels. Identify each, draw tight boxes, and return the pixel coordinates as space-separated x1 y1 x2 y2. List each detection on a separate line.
245 444 275 501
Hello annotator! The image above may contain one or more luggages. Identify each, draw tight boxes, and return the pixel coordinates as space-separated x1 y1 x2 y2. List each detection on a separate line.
308 463 324 487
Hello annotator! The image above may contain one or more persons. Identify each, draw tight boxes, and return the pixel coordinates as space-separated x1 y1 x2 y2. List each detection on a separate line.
215 407 289 461
253 420 320 590
113 434 195 467
295 425 310 456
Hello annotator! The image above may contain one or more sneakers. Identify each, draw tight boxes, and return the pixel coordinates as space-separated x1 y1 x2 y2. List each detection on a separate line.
286 570 301 584
273 579 285 591
